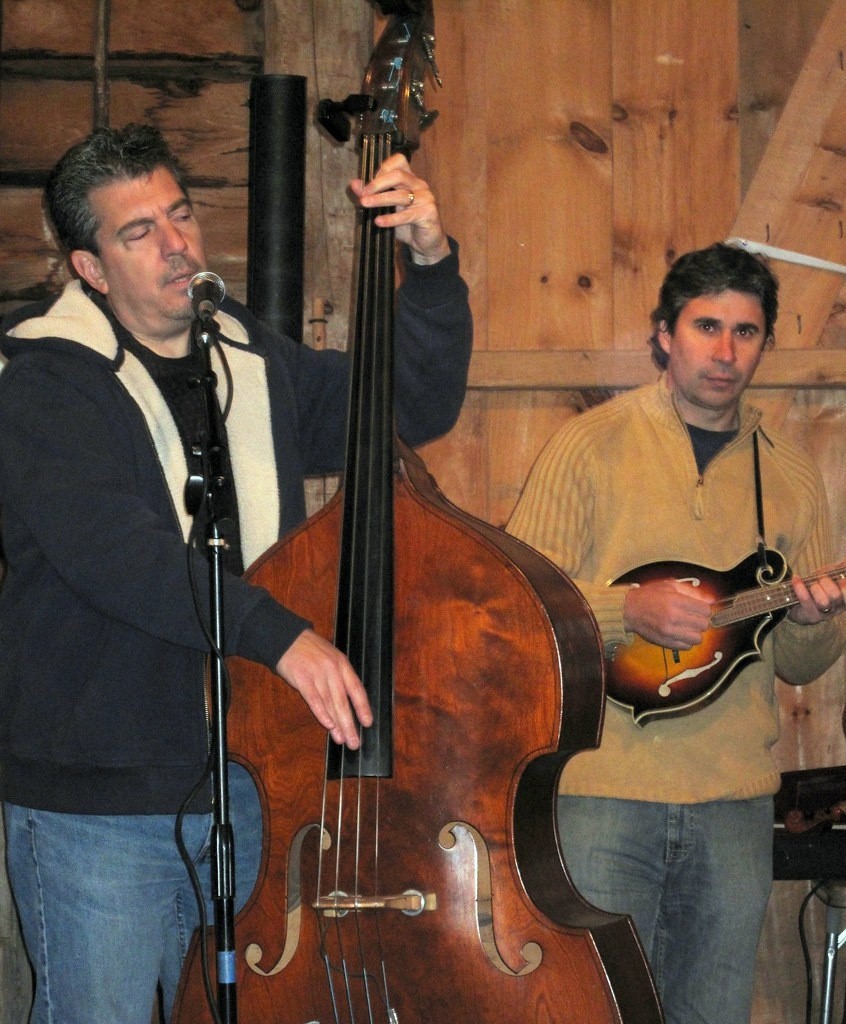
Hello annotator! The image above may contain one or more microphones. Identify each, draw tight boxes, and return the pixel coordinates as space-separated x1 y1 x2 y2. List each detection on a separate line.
187 272 225 321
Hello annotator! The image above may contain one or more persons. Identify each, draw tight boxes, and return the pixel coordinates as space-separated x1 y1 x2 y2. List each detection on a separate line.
503 243 846 1024
0 118 475 1024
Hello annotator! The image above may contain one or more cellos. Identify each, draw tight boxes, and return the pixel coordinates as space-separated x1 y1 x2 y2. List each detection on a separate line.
172 0 663 1023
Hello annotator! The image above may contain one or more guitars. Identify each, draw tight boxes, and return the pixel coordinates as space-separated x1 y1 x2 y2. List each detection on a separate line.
601 545 845 730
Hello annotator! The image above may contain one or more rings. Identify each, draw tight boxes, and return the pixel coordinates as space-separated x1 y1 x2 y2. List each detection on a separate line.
818 608 830 613
405 189 414 206
